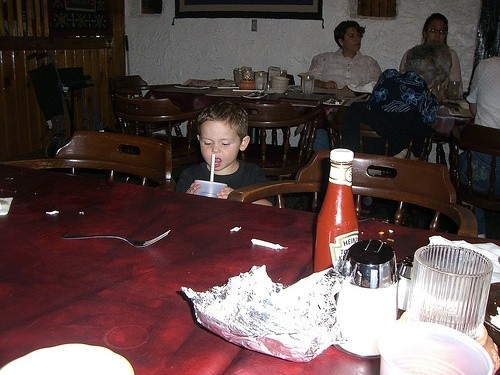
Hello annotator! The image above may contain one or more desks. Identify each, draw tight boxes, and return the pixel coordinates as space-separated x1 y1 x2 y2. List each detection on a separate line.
0 164 500 375
139 84 370 149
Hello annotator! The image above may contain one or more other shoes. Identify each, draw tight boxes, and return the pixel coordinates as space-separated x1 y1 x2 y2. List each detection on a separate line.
361 198 375 214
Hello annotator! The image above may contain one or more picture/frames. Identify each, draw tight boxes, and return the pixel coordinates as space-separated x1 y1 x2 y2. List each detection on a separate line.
171 0 324 29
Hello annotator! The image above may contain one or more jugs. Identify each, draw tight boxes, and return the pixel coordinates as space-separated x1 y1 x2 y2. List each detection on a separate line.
431 67 449 102
404 244 493 341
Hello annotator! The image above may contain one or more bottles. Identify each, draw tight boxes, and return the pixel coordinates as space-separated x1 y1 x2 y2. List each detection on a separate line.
398 254 414 310
335 239 399 361
314 148 359 274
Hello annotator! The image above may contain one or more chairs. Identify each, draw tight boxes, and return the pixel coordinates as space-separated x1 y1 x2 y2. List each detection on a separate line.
0 72 500 238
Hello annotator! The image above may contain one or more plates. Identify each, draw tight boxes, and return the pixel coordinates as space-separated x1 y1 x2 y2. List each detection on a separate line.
243 92 267 99
233 89 264 92
174 85 210 89
483 282 500 333
323 98 345 105
217 87 239 89
0 344 136 375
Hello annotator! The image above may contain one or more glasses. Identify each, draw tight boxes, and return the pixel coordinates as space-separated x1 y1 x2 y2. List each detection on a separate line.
430 28 447 34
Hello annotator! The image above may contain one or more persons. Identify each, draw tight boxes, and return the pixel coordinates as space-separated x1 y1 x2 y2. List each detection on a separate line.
400 13 462 163
342 41 452 158
175 98 276 207
307 20 382 151
458 51 500 196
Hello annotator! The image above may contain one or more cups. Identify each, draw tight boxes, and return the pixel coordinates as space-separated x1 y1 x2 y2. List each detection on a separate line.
377 320 494 375
194 181 227 197
448 81 461 99
302 75 315 95
234 66 290 94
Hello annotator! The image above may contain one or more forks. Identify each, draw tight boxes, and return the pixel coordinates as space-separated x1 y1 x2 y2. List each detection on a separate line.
62 229 171 248
